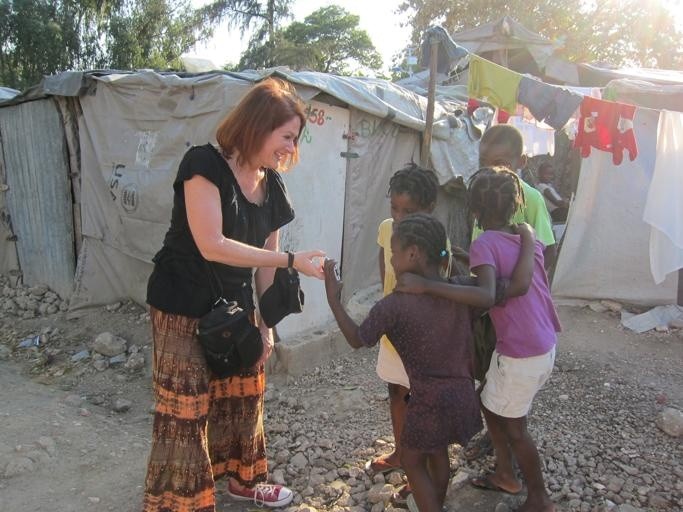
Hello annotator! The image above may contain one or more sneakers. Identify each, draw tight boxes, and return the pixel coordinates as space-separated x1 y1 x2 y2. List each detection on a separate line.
226 479 294 508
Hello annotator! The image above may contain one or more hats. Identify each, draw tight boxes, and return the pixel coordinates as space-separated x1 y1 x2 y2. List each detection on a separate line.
258 250 305 329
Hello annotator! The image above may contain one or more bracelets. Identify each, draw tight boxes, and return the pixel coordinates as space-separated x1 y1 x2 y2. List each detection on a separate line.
285 250 294 268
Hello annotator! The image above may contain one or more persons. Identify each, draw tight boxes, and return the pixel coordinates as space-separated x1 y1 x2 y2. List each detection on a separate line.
537 163 570 221
392 164 562 512
452 125 557 470
144 80 327 512
324 215 536 512
365 163 453 508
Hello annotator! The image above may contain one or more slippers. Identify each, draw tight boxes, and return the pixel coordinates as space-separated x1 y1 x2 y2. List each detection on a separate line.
468 470 523 493
365 453 402 473
460 431 492 461
393 482 412 504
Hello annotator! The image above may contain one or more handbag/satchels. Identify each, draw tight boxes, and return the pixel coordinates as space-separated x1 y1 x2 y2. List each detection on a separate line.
195 300 264 379
